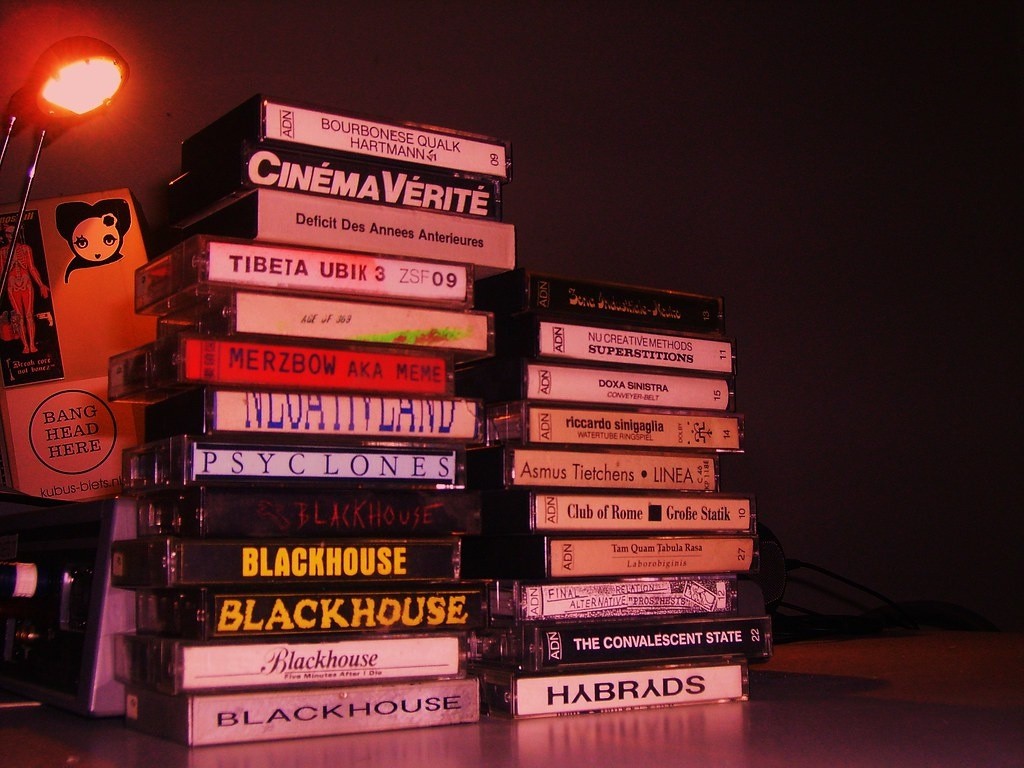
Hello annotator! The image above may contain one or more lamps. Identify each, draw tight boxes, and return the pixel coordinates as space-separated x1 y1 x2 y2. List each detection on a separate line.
0 36 129 296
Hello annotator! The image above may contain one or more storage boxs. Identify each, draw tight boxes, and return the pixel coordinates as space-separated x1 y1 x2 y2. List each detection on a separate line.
105 92 772 748
